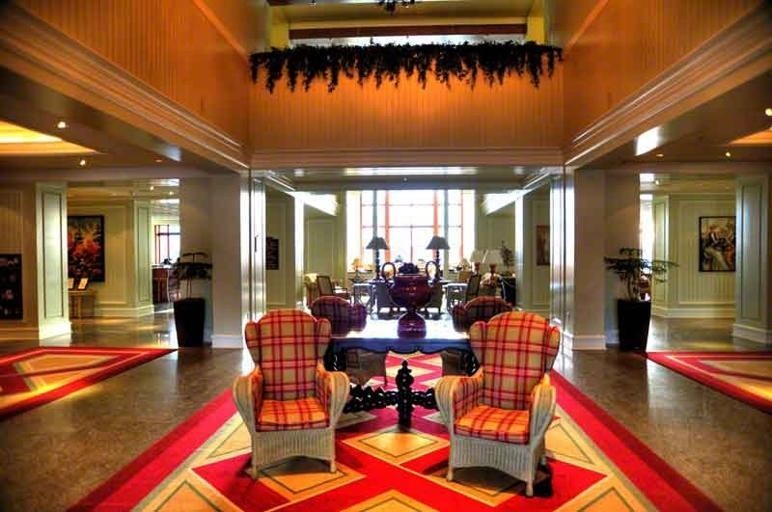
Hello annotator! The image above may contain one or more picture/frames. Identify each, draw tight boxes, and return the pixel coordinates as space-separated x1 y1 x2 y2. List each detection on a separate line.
68 215 105 283
68 277 74 288
698 216 736 272
535 223 550 265
78 278 88 289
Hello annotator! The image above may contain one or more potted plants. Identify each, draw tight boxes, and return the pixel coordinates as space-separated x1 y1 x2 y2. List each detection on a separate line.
172 252 213 347
604 247 679 352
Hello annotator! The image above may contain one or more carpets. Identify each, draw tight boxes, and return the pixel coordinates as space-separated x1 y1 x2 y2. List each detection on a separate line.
63 340 723 512
638 351 772 415
0 346 176 420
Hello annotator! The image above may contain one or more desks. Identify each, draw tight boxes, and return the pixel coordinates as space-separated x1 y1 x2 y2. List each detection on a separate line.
330 318 482 427
69 289 96 319
153 269 169 304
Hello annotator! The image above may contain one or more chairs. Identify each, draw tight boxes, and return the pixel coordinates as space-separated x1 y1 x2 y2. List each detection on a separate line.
233 306 350 475
433 308 560 496
303 271 519 328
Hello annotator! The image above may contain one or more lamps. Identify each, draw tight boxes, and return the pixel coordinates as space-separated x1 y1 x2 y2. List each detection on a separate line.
483 250 504 276
469 250 486 278
426 235 450 279
459 258 468 271
366 236 389 279
351 258 362 278
